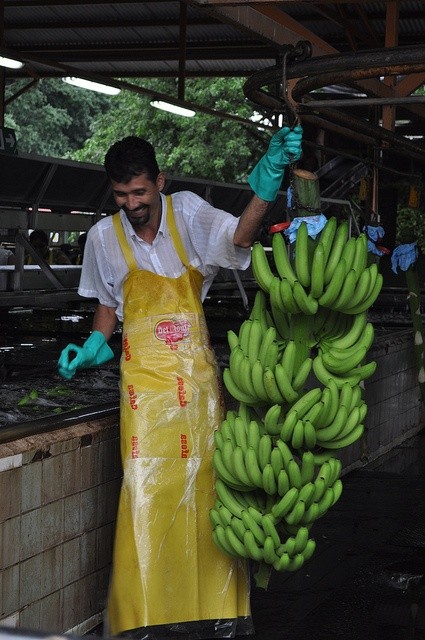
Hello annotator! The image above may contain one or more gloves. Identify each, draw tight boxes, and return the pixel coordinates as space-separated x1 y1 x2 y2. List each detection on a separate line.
247 126 303 201
58 331 114 380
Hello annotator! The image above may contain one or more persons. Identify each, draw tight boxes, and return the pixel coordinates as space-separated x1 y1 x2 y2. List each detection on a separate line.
58 125 303 640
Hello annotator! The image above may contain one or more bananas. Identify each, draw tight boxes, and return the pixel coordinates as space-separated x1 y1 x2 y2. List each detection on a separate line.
209 216 384 573
